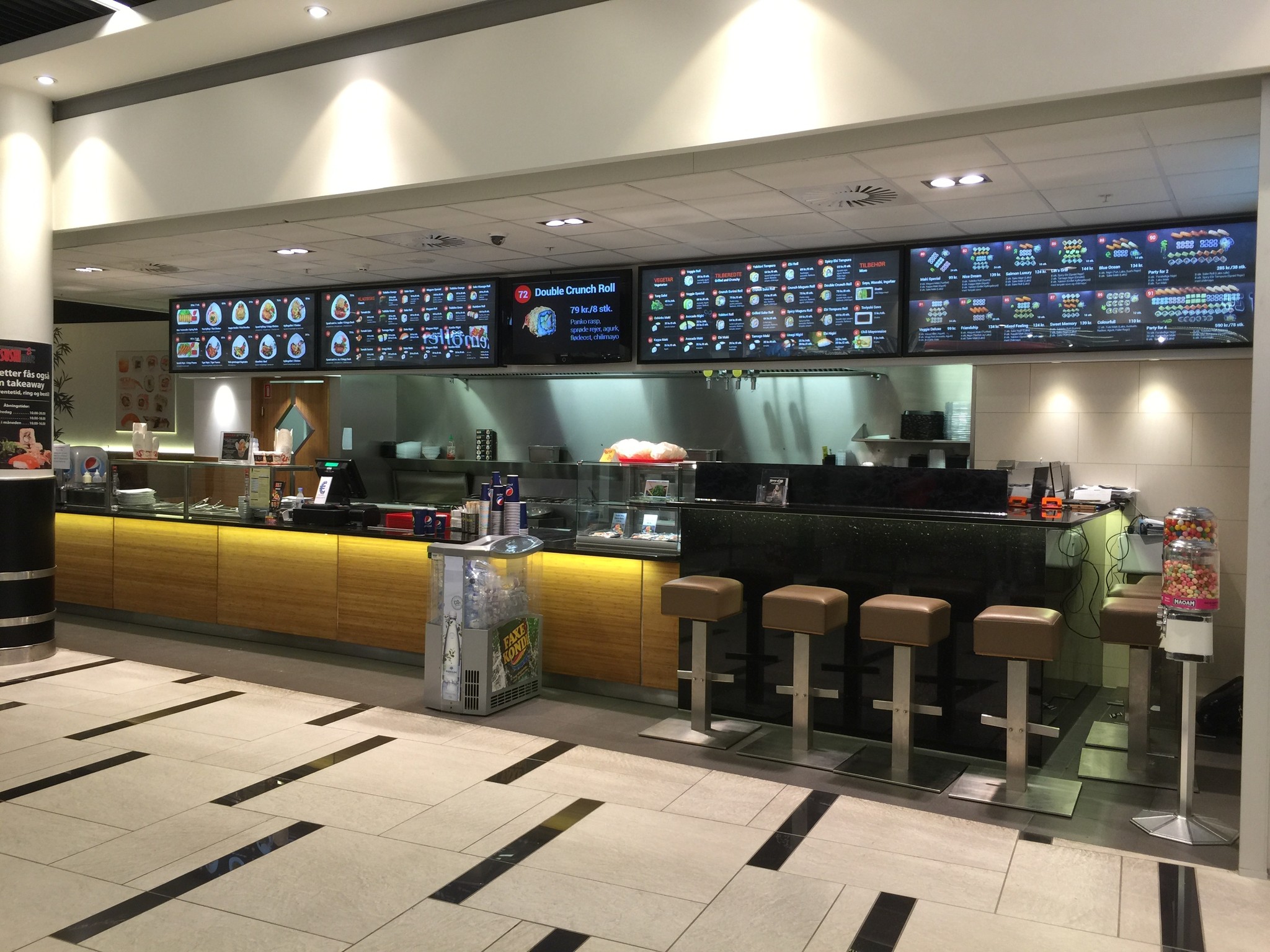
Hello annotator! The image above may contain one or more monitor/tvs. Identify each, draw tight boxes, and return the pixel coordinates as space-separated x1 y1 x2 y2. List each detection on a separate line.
499 268 633 366
314 457 369 504
637 245 904 365
318 276 498 371
902 214 1257 356
169 289 319 373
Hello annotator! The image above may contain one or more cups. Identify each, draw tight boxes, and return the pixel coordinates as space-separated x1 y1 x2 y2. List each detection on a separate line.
426 507 436 535
447 446 456 460
411 506 428 536
435 515 448 532
479 471 529 541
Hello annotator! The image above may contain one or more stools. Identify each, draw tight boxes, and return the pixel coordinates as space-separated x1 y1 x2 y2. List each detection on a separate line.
949 604 1083 819
832 594 971 794
637 575 763 752
1077 573 1218 793
737 583 869 772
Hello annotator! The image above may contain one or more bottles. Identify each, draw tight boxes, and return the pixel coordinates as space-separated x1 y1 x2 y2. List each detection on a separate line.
102 471 107 483
92 469 103 483
296 487 304 509
82 471 92 483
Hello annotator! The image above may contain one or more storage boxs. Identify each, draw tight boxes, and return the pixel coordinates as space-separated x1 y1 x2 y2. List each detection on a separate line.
685 448 721 461
528 445 560 462
252 438 284 465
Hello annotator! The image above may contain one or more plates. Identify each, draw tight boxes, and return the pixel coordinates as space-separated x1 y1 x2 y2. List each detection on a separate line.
115 486 157 506
238 493 313 519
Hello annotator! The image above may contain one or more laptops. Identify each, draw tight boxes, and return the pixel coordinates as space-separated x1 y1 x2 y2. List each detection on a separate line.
1048 461 1110 505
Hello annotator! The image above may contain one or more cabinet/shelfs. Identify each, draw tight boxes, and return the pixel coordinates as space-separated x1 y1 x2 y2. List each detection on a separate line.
575 460 697 555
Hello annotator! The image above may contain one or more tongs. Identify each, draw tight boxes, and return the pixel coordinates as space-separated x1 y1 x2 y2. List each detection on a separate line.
192 496 211 507
210 499 225 509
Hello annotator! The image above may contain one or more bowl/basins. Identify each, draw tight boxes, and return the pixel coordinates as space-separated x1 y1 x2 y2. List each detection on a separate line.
421 445 441 459
